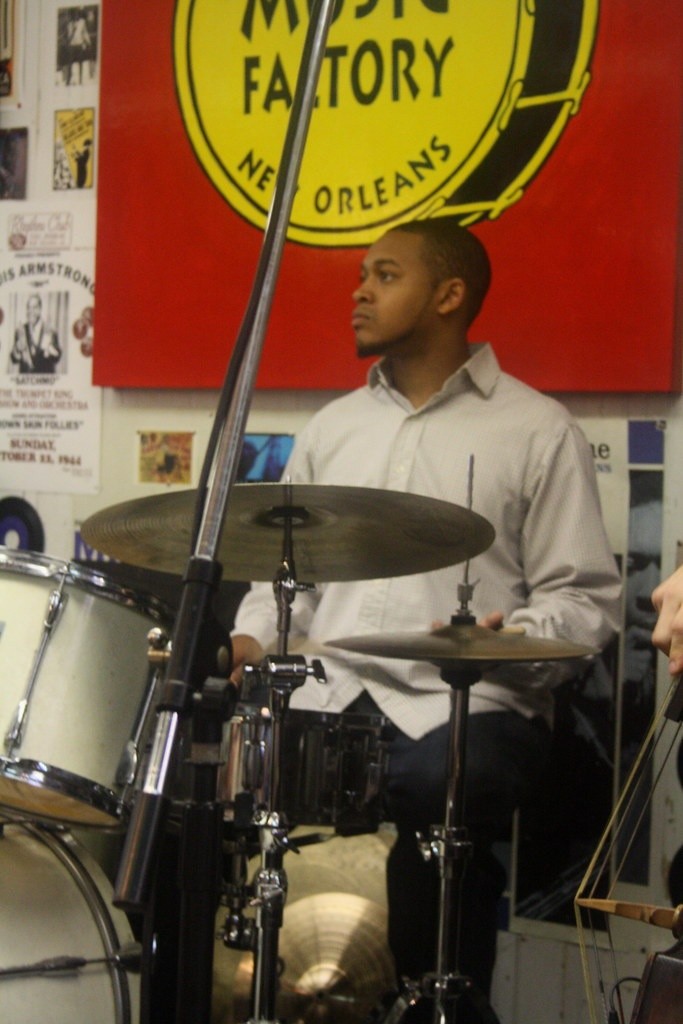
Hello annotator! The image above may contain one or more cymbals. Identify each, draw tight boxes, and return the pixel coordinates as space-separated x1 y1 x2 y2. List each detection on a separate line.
77 474 496 586
326 620 604 668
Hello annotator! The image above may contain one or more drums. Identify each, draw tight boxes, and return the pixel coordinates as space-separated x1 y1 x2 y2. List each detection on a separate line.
0 543 178 833
0 804 150 1024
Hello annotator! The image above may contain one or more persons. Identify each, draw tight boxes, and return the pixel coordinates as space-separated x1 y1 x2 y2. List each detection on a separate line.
137 216 626 1024
7 292 64 377
59 7 93 86
646 561 682 946
